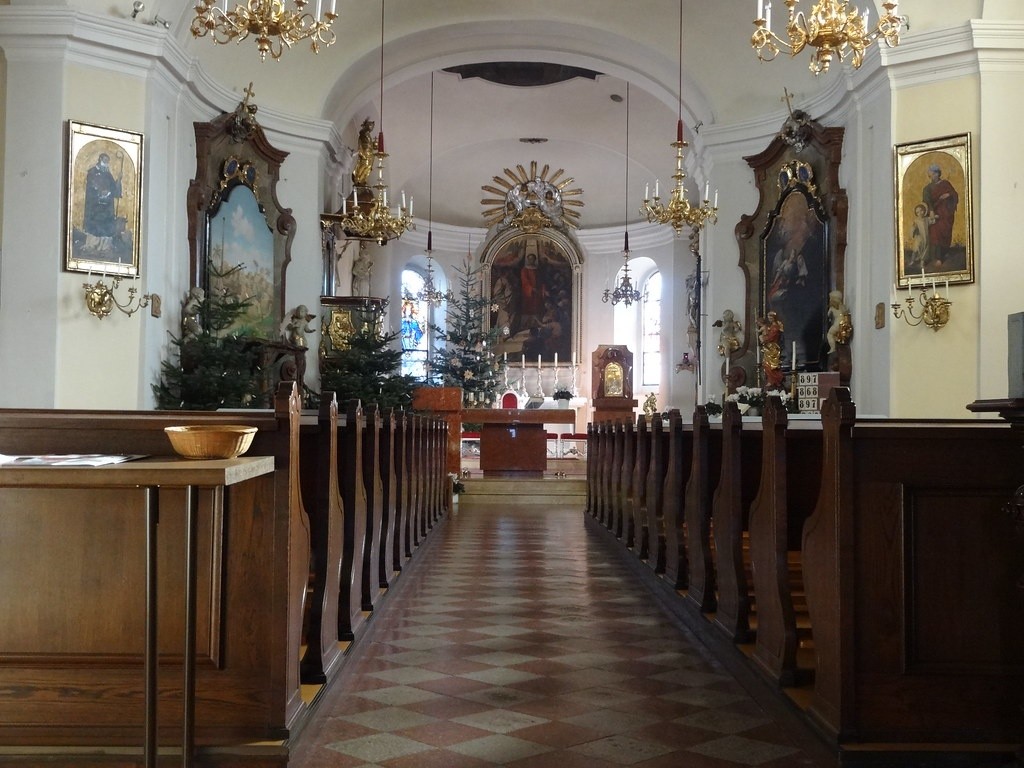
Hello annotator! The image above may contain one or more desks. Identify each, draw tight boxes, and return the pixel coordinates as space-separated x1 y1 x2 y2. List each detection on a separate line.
0 455 277 768
462 408 575 479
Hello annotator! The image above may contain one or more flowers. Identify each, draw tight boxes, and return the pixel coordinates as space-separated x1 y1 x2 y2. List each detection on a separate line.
766 390 795 406
724 385 764 407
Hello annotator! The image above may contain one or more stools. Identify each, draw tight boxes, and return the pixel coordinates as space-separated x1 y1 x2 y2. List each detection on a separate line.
546 433 559 460
461 432 480 441
561 433 588 459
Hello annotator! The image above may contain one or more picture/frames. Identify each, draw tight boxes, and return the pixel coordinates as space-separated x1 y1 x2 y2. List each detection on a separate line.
481 226 582 370
202 154 281 341
759 158 829 372
893 132 975 290
63 118 145 281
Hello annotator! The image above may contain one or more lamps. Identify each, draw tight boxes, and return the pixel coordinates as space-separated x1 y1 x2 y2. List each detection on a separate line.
639 0 720 238
602 81 649 309
890 266 954 334
83 256 151 321
399 71 459 309
189 0 339 65
750 0 912 77
340 0 418 242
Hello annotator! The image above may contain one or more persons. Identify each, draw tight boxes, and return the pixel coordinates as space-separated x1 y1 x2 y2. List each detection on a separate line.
720 309 739 357
351 121 375 184
759 311 783 386
181 288 206 344
288 305 316 349
827 291 849 353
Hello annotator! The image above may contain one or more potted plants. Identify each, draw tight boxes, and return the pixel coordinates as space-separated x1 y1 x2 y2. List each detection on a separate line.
553 390 574 408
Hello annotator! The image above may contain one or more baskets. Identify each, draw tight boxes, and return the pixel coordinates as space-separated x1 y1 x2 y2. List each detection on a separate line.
164 426 260 459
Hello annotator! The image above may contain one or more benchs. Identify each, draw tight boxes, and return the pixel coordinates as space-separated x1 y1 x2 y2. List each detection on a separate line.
584 384 1024 768
0 378 452 768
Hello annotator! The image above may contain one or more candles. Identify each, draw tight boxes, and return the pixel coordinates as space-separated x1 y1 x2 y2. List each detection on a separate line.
521 354 525 368
573 351 577 367
503 351 507 364
756 345 760 364
726 357 730 375
554 352 558 367
537 353 542 369
792 341 796 371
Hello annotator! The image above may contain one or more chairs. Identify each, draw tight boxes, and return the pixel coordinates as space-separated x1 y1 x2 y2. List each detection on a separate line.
501 391 520 409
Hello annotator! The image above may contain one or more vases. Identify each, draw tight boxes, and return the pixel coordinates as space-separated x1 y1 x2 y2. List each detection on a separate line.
742 406 761 417
